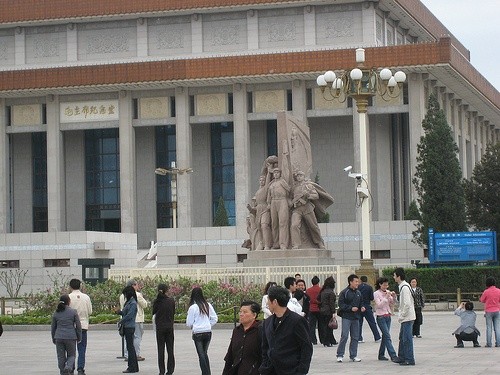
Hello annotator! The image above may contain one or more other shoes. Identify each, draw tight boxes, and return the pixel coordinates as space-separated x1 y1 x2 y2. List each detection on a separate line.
378 355 388 361
123 368 138 373
125 356 145 361
395 358 416 365
374 338 382 342
474 343 481 347
453 344 463 348
357 340 364 343
77 368 86 375
392 357 397 361
413 335 422 338
485 345 500 347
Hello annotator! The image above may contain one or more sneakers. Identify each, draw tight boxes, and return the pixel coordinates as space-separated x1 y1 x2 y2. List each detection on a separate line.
337 356 343 362
353 357 362 361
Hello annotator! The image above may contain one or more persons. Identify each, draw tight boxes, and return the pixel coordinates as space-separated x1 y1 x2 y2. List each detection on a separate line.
262 274 310 320
336 274 366 362
392 268 415 366
452 301 481 347
152 284 175 375
306 276 338 346
409 278 424 338
68 279 92 375
355 276 381 342
259 285 313 375
222 300 263 375
114 280 147 373
245 155 326 250
51 295 82 375
480 278 500 346
186 288 218 375
372 278 398 362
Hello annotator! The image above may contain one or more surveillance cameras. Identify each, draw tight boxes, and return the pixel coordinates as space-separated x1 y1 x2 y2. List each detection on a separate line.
343 166 353 172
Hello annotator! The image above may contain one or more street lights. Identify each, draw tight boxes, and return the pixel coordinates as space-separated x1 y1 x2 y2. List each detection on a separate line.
314 47 407 292
155 162 193 228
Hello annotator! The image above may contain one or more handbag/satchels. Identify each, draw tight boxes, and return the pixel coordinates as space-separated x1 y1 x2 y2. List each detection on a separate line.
415 307 423 325
119 325 124 336
327 314 337 329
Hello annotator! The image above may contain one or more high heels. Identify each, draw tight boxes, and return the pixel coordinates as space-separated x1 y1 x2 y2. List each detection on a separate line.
323 342 338 347
